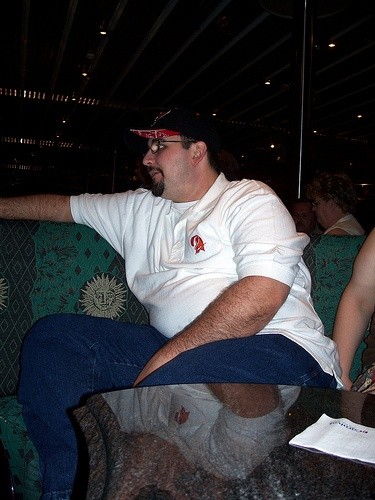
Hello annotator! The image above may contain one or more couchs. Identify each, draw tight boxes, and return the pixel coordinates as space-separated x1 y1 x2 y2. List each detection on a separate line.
0 223 375 500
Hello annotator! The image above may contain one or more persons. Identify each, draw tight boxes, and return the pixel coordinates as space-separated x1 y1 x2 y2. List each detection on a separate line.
102 384 302 500
0 109 344 500
288 197 324 234
308 172 365 235
333 227 375 393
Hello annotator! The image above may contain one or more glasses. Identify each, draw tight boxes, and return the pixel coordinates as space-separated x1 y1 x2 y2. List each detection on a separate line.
141 139 197 157
313 199 322 207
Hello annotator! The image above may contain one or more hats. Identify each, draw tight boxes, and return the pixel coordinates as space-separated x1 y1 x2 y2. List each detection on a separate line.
129 104 220 152
